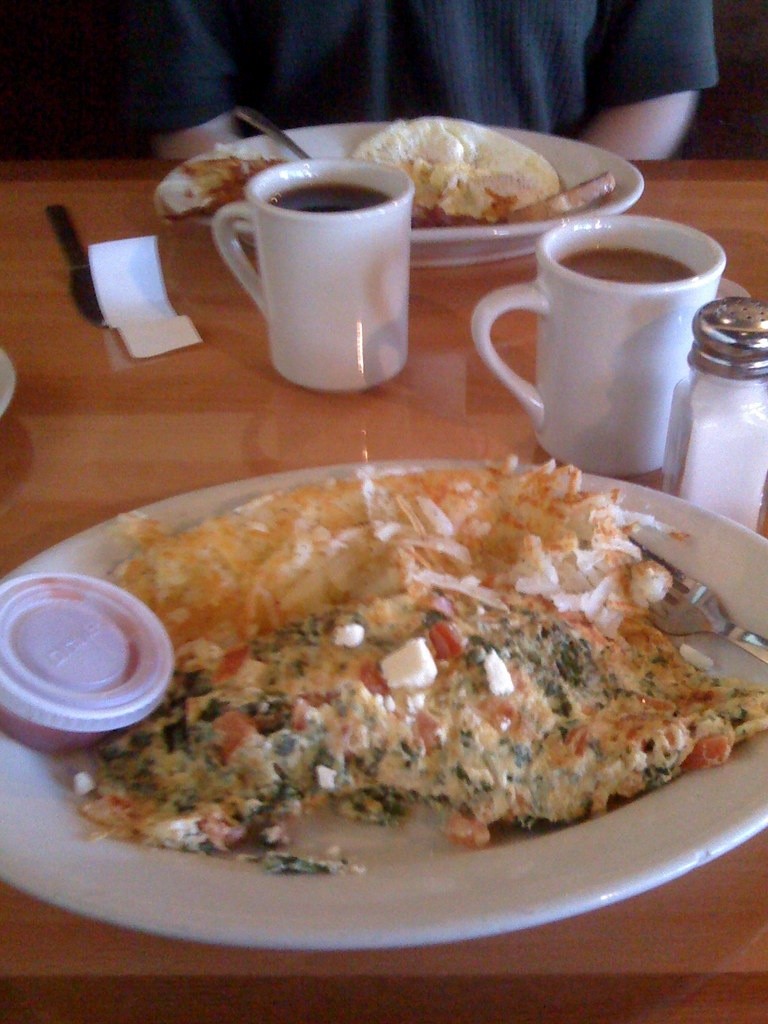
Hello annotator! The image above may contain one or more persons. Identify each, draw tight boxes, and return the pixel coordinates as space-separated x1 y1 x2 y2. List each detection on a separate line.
111 0 720 162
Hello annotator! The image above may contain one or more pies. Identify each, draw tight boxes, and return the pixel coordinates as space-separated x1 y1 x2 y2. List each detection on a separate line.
72 463 768 858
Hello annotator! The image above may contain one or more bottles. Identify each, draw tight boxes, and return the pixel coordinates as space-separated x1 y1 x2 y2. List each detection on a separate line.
662 298 768 533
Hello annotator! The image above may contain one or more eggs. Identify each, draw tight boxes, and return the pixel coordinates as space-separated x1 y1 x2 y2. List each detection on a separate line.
352 116 563 219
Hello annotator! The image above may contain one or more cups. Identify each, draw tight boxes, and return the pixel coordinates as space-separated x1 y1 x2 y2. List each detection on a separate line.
212 158 414 392
472 217 727 478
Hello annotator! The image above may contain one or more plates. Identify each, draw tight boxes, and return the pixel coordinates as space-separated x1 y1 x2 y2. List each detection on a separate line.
0 351 15 419
0 460 768 949
158 122 644 268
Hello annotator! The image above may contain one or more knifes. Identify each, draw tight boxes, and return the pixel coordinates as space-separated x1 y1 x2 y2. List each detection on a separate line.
45 205 108 328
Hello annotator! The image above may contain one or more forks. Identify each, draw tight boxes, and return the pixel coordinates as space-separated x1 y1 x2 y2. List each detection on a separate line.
628 535 768 665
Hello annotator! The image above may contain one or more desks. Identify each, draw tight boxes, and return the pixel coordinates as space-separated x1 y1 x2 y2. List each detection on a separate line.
0 157 768 1024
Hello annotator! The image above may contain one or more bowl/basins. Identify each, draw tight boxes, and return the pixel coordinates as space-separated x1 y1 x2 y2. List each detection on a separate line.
0 572 176 751
